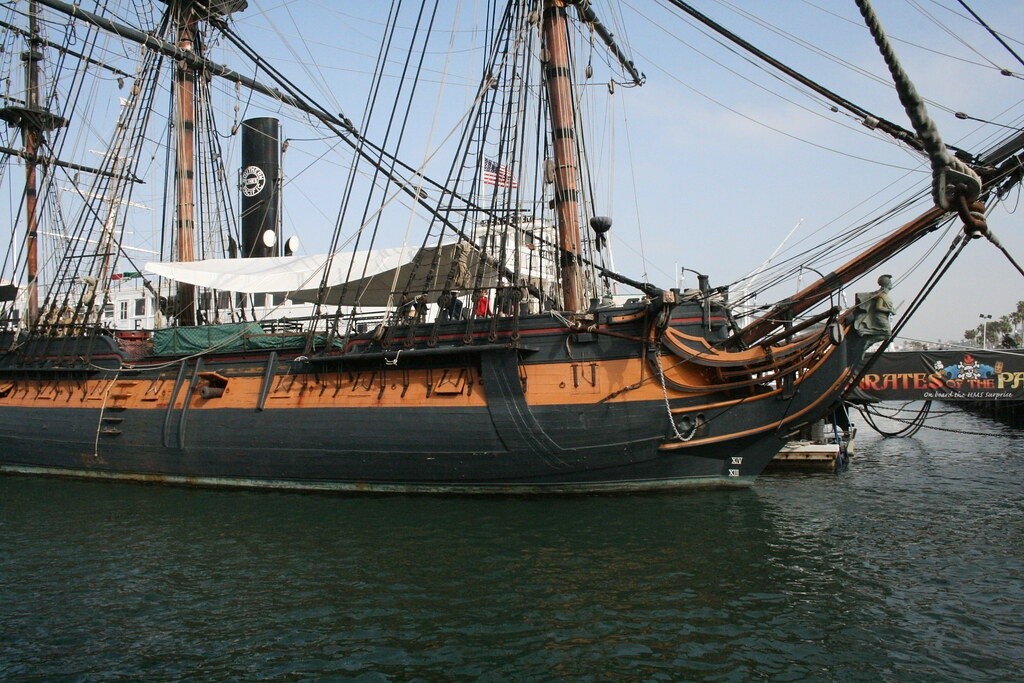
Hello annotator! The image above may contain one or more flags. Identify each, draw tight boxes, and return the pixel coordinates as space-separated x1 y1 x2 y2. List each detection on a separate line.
485 159 519 188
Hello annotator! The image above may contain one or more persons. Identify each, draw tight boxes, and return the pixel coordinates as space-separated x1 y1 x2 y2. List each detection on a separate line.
853 275 896 361
475 289 492 318
401 293 463 323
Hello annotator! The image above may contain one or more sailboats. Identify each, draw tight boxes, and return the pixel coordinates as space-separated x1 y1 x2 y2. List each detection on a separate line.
1 1 1024 498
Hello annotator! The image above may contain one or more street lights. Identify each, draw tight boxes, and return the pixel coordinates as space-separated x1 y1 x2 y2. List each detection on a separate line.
980 314 992 349
